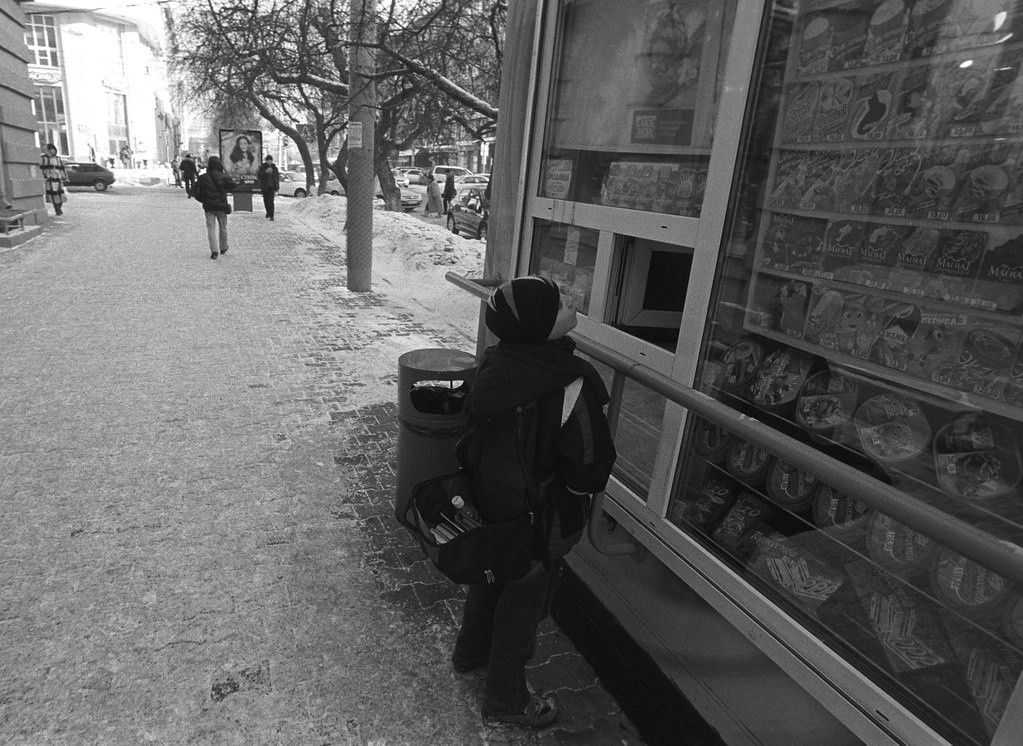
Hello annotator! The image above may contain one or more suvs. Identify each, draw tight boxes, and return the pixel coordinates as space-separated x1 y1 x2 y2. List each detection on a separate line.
432 165 473 183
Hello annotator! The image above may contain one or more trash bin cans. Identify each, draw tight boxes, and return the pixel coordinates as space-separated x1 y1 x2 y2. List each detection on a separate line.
392 346 481 532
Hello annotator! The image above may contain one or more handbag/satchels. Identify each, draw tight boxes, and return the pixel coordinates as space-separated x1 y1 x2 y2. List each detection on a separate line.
225 204 231 214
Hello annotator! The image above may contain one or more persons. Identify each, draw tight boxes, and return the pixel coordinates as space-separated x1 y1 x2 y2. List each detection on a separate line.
191 156 236 259
180 154 196 198
194 157 203 178
125 144 134 168
483 165 493 200
257 155 279 221
120 147 130 168
449 276 617 729
171 155 184 188
40 144 69 216
204 150 209 163
421 174 443 218
229 136 259 175
442 169 455 214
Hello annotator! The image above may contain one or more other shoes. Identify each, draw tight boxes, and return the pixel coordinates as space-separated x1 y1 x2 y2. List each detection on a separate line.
271 216 274 220
266 214 269 218
211 252 218 259
221 245 228 254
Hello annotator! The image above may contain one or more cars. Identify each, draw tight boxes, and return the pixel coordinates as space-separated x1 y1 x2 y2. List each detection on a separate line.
62 161 116 191
278 171 307 198
288 160 300 170
419 167 433 185
446 187 489 240
438 176 489 196
296 164 318 182
325 175 423 211
397 167 420 183
390 169 409 188
454 174 490 182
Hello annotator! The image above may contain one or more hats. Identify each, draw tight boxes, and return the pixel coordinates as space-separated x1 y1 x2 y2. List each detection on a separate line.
483 274 560 345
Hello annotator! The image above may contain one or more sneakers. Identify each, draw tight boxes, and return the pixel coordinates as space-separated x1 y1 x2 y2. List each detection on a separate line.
480 693 558 728
450 662 490 679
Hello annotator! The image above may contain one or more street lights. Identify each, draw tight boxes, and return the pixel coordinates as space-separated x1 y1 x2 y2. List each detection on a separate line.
162 128 170 185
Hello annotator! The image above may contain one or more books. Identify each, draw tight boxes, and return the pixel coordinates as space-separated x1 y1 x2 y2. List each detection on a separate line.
430 511 482 546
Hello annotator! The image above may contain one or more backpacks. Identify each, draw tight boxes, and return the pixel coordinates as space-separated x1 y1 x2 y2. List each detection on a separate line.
394 401 564 584
